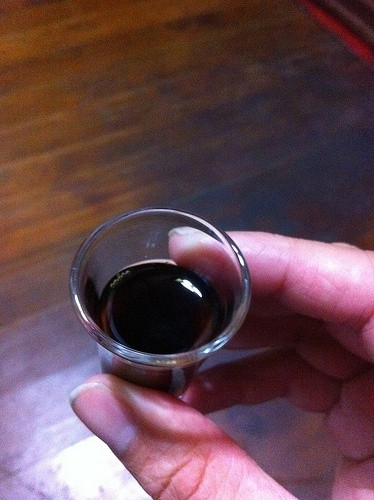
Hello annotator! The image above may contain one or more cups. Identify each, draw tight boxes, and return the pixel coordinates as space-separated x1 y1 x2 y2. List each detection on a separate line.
69 205 252 397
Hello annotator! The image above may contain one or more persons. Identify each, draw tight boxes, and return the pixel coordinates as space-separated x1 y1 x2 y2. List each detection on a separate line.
70 225 373 500
